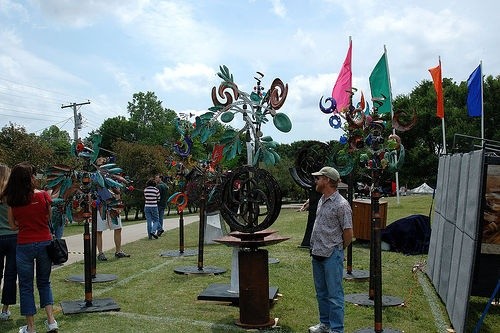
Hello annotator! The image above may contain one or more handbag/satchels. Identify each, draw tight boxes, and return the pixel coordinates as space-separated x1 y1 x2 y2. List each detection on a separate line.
50 239 68 265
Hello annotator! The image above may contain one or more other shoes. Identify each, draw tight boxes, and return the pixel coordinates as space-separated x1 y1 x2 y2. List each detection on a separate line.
150 232 158 239
157 230 164 236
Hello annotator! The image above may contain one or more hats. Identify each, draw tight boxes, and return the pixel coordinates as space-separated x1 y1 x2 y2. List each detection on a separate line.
311 167 340 181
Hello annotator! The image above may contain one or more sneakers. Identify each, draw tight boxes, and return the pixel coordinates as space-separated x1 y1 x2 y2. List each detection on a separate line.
45 319 59 333
308 322 329 333
0 310 11 321
18 325 29 333
98 253 107 261
329 330 339 333
115 250 130 257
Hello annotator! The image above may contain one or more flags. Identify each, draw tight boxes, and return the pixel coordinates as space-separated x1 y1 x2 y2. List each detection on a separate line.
428 56 443 119
332 37 351 113
369 45 392 115
466 60 484 116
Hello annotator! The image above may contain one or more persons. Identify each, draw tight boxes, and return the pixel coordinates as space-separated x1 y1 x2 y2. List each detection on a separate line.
97 209 130 260
0 164 19 321
155 176 169 236
0 161 59 333
143 179 160 239
309 167 353 333
